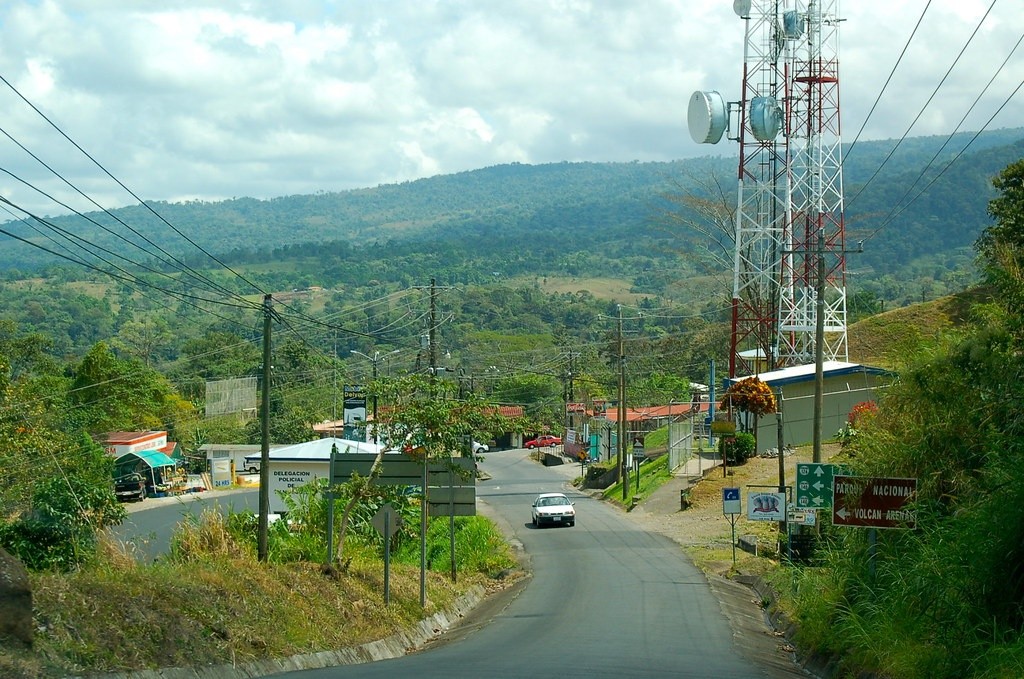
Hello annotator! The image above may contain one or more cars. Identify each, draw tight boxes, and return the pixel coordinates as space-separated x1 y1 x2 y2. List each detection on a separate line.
115 474 146 502
524 434 562 450
473 440 489 454
530 492 577 529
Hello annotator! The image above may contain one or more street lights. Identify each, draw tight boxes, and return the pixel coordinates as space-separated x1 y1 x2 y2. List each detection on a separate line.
349 349 400 444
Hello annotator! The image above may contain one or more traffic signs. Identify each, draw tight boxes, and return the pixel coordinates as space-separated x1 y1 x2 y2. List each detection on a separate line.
795 462 833 509
832 474 918 530
